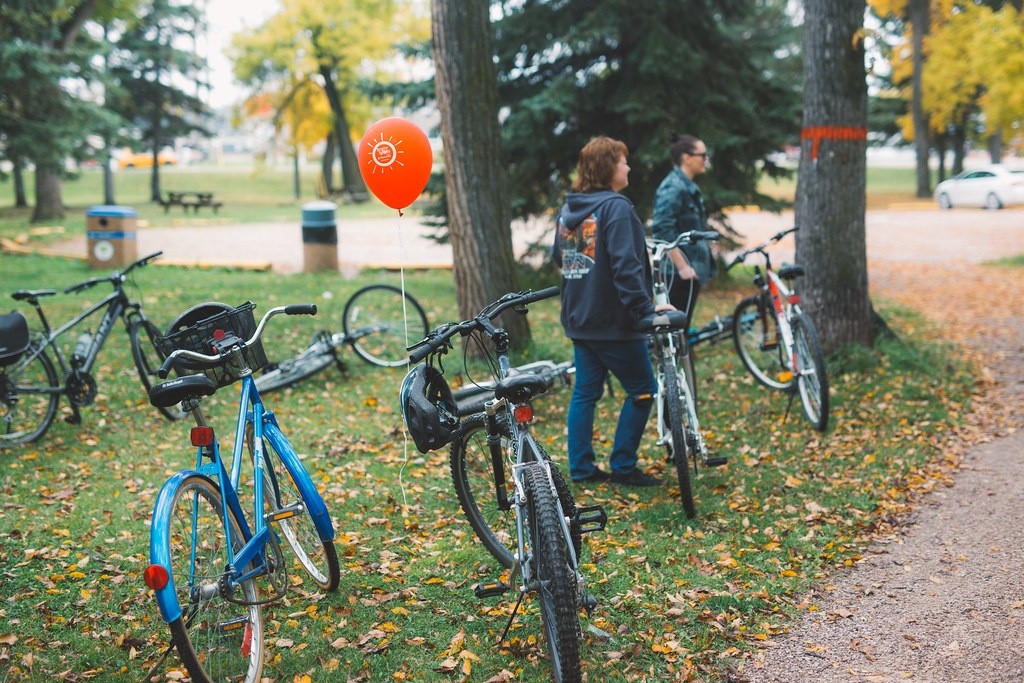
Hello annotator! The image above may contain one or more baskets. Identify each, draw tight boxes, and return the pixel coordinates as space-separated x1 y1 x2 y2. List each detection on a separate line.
153 300 269 397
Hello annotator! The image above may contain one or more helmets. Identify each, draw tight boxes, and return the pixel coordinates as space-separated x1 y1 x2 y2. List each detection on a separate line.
399 362 460 454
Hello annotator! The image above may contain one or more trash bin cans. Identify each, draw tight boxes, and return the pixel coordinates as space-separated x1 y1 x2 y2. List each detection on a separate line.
302 200 340 274
85 205 138 271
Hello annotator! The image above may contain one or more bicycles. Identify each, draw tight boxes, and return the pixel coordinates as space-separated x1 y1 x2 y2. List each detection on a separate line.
646 231 720 519
668 309 770 346
406 285 608 683
254 284 430 394
143 300 340 683
725 226 830 431
0 250 190 450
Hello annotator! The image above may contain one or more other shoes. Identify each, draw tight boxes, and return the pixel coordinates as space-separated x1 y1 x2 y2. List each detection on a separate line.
611 470 660 486
573 466 611 481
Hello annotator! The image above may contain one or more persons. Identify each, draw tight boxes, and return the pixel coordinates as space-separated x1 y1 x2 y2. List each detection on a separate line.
552 136 664 488
652 130 717 337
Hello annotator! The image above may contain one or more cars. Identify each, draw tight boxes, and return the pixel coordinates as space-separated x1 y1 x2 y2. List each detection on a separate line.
117 147 177 168
934 164 1024 209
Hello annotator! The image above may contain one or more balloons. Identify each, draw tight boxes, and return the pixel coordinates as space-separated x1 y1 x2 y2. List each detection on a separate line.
357 116 433 216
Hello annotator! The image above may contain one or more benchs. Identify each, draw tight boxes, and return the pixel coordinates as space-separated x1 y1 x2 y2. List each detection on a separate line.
156 190 222 216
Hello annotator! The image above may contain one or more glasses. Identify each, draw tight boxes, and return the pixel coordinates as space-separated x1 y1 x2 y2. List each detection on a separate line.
686 150 706 159
